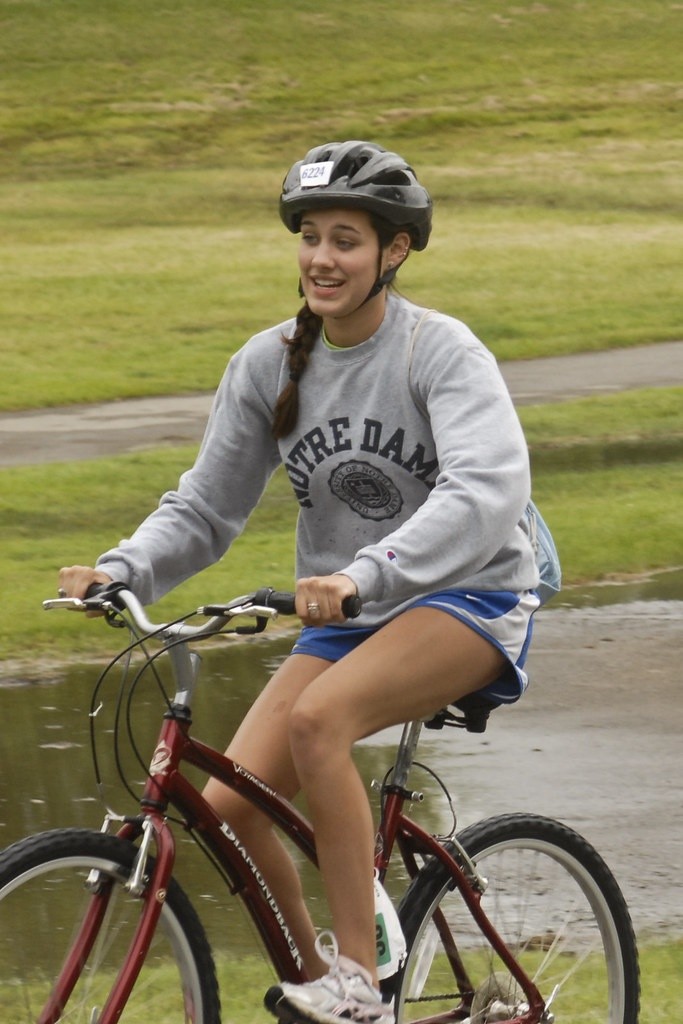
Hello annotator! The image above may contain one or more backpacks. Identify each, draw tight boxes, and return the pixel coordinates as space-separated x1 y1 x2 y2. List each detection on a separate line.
275 308 562 610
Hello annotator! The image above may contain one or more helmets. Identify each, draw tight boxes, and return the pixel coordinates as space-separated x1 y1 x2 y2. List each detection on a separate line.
278 138 434 253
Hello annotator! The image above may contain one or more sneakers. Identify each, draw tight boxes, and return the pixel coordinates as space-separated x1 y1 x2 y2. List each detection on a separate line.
263 966 397 1024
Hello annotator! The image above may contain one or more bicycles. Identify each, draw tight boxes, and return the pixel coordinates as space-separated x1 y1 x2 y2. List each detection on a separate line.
1 580 641 1024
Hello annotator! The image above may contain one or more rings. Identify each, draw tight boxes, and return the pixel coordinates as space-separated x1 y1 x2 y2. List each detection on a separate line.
307 602 319 612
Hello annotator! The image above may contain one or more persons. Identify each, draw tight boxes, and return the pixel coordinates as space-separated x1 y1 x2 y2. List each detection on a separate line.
58 136 543 1024
